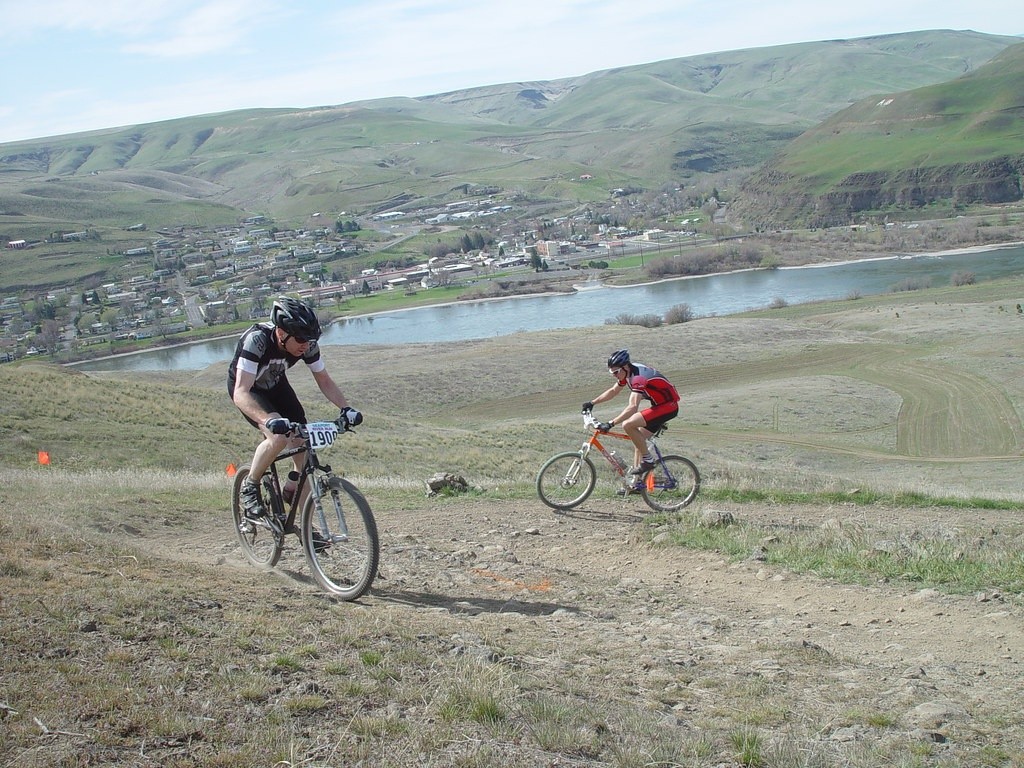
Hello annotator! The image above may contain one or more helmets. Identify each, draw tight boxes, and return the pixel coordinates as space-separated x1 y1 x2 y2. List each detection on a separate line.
608 349 630 367
270 296 323 341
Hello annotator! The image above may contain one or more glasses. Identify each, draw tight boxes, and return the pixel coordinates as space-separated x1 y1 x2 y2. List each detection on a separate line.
609 367 623 375
294 336 311 344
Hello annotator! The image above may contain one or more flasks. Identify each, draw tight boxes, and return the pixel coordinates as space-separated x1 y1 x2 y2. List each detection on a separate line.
610 452 628 470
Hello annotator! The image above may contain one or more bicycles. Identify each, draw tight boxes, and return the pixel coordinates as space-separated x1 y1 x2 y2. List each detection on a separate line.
230 412 381 602
535 405 702 513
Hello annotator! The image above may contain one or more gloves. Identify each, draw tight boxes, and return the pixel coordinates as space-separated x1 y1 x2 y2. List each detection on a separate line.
264 417 293 435
340 406 363 427
580 401 594 414
596 420 615 431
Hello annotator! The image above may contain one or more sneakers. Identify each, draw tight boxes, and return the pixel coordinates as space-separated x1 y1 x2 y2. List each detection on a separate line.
299 526 330 551
631 461 656 474
240 480 268 520
617 488 641 495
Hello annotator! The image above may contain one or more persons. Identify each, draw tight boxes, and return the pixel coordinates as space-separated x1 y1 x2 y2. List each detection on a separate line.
227 295 363 551
581 349 680 495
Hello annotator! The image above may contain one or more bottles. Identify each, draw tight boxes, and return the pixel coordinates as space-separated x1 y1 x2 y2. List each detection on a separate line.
282 470 299 503
